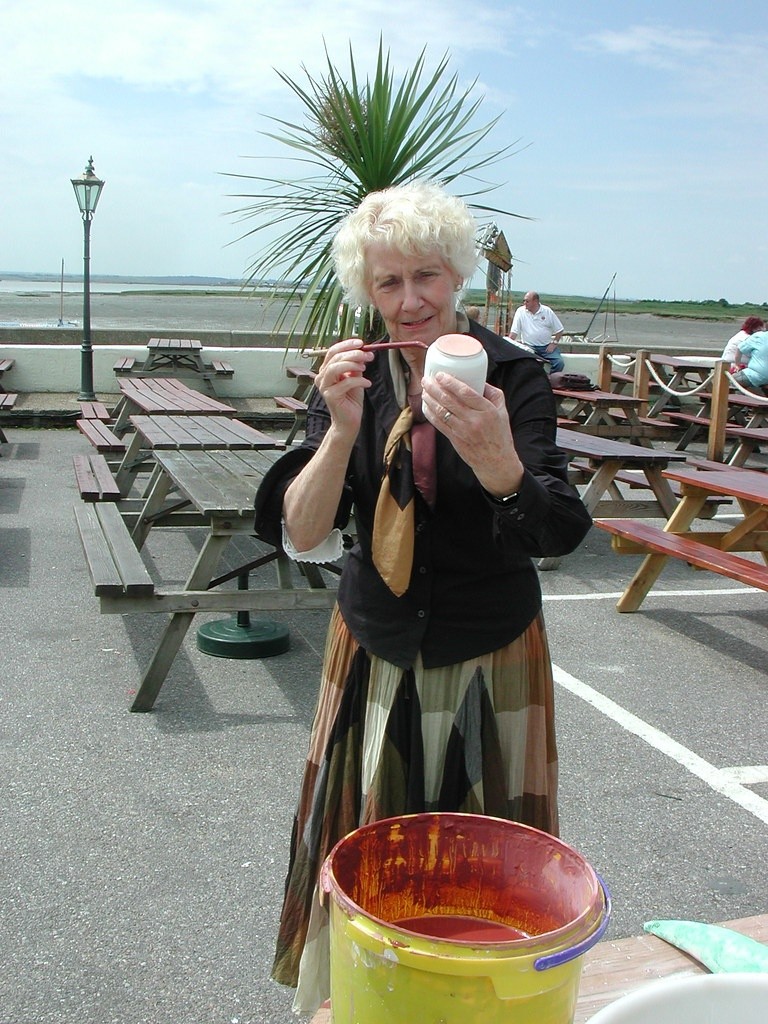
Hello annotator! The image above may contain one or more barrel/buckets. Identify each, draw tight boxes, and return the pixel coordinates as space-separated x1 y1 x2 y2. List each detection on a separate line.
318 812 612 1024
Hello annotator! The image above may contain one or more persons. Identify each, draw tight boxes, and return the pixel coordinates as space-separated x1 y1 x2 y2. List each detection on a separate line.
721 317 766 398
255 183 593 1016
731 318 768 388
465 306 480 323
509 291 565 374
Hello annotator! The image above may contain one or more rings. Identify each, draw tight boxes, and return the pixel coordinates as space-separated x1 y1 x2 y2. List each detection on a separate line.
443 411 451 422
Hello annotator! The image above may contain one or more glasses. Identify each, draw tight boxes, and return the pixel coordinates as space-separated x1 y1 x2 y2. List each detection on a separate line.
523 299 534 302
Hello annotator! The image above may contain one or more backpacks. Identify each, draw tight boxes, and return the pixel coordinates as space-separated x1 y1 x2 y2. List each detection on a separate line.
548 371 601 391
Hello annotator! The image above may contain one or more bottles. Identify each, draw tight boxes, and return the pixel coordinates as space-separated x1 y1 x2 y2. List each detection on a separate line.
422 334 488 415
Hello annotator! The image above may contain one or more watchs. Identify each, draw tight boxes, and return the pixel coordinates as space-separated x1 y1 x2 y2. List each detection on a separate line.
493 489 520 506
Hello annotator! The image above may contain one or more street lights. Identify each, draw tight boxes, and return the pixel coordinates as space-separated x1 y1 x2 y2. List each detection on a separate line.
70 156 105 402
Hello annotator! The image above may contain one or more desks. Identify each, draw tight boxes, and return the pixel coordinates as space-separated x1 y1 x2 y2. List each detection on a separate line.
536 353 768 614
114 415 287 512
309 914 768 1024
109 378 191 441
136 337 220 401
112 389 238 461
127 448 339 712
284 366 317 446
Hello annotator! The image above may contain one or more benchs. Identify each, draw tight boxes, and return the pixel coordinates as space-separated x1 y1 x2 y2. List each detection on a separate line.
659 411 744 439
684 458 742 472
112 357 143 378
71 501 186 615
76 417 128 461
0 393 19 444
72 453 144 513
566 461 733 520
611 370 665 395
79 401 117 425
273 396 308 421
557 417 586 434
607 411 678 438
205 361 235 380
0 359 15 394
668 372 740 395
591 517 768 613
